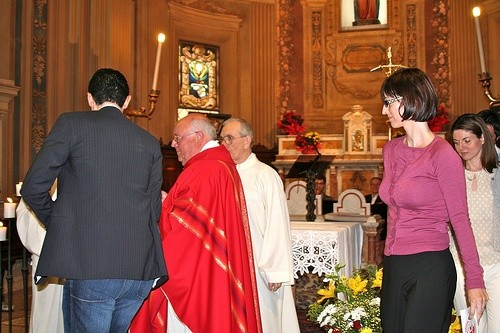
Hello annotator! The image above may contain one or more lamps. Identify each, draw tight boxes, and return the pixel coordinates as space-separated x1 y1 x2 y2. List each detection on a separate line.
0 221 7 241
151 32 165 91
15 181 23 196
4 197 16 219
472 6 486 72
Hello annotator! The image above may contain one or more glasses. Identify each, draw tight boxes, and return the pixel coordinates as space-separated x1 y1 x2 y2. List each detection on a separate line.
382 96 404 107
371 183 380 188
172 133 195 142
217 135 247 145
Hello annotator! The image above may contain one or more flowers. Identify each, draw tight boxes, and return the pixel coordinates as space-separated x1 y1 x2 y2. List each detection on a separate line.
277 111 307 135
428 0 452 131
295 131 322 155
305 263 383 332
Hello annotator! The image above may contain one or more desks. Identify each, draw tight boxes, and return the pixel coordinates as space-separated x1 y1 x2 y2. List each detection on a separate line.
290 221 364 309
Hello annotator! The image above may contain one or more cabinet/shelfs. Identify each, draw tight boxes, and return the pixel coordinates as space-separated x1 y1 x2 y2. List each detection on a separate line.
270 133 385 201
160 146 271 193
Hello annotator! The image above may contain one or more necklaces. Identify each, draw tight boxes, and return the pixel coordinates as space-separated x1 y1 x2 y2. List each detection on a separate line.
465 164 485 180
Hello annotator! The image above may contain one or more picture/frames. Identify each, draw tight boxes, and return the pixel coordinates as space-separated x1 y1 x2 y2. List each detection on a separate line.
337 0 389 32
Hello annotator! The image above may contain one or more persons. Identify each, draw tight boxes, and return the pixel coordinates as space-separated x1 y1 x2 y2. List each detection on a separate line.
312 176 334 198
15 177 67 332
216 118 303 332
19 68 170 332
130 113 264 333
474 109 500 167
449 110 499 333
364 176 385 205
377 68 489 333
